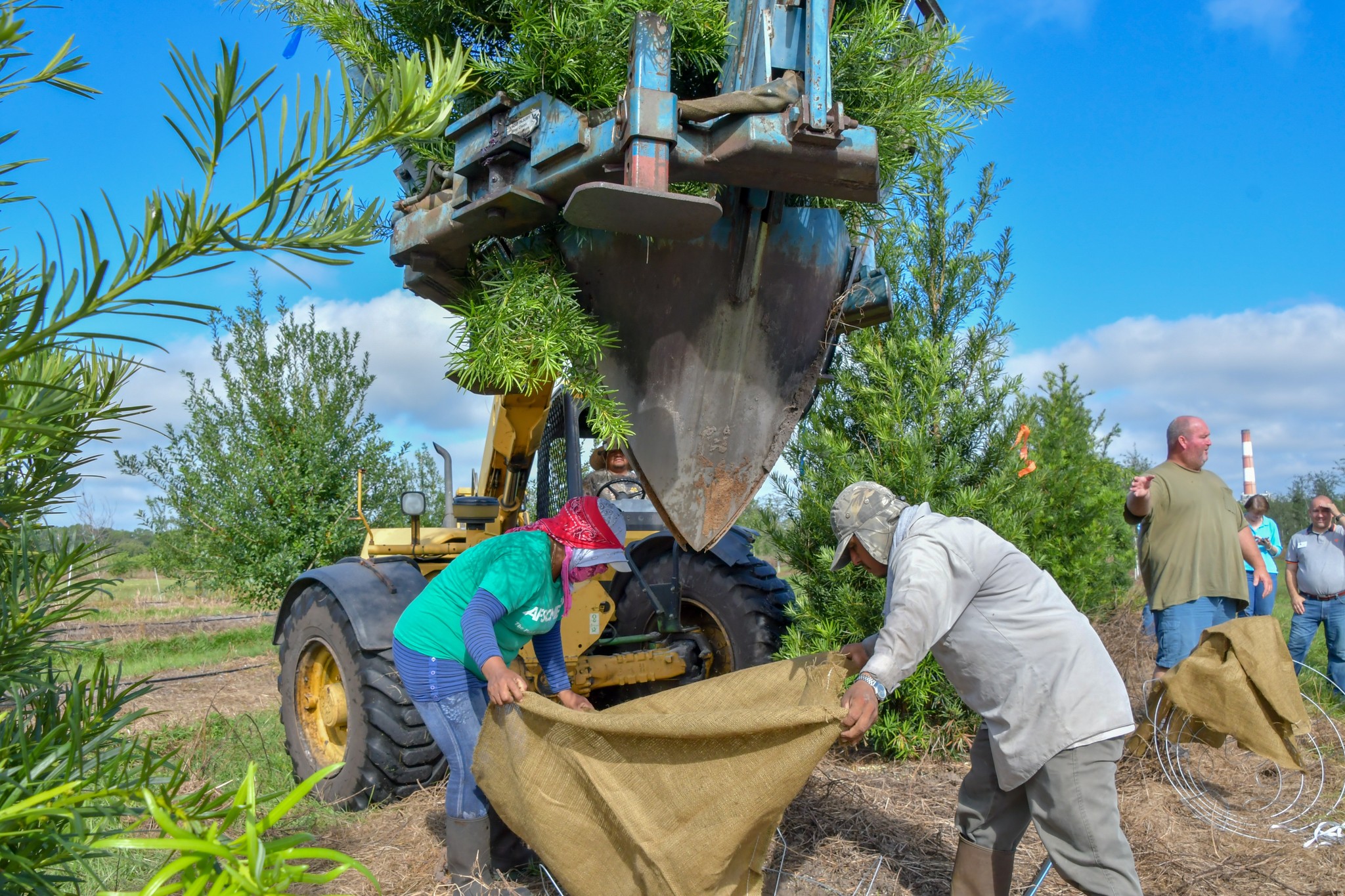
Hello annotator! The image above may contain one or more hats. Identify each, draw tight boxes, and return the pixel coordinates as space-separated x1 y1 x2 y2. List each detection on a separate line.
568 497 633 573
829 483 912 572
589 431 634 471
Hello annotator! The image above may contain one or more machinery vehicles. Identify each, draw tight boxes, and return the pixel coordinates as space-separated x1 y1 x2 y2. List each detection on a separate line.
274 0 898 812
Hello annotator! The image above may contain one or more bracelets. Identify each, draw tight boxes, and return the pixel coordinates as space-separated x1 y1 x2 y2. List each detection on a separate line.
1265 543 1272 551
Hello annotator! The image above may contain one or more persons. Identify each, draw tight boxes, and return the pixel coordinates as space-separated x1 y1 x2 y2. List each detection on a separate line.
393 495 633 896
1124 415 1273 694
829 480 1145 896
1237 493 1283 618
582 433 649 500
1285 495 1345 702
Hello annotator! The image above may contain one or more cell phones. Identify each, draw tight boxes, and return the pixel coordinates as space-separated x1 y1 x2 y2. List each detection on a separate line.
1262 537 1268 543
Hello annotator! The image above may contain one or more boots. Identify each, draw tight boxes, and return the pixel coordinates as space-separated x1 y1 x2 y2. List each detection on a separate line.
445 811 533 896
949 834 1016 896
486 804 544 883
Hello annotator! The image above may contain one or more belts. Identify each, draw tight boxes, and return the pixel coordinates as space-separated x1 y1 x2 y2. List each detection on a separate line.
1300 590 1345 601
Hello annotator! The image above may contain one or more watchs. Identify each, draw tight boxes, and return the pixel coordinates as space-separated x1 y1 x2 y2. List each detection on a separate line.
852 674 886 706
1335 512 1345 521
